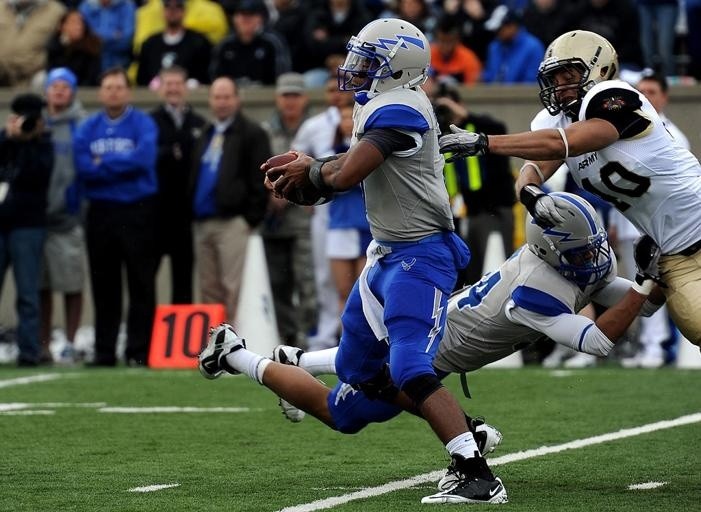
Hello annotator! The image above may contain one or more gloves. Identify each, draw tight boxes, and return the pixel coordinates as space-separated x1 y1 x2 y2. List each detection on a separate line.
631 235 662 286
520 182 566 229
438 124 490 164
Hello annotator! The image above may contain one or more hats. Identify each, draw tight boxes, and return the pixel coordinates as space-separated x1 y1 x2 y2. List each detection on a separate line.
276 73 309 97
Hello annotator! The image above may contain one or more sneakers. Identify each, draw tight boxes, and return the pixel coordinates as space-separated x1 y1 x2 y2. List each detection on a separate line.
272 344 307 422
421 418 510 504
197 323 247 380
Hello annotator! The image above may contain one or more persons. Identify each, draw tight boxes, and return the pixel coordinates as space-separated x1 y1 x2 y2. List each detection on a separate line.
199 190 667 434
266 16 512 504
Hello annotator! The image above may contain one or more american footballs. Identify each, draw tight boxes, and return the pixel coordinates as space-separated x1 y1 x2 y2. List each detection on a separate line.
265 154 333 207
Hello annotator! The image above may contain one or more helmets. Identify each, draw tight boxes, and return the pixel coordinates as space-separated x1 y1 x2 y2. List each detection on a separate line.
525 191 613 287
537 30 620 118
336 17 431 106
